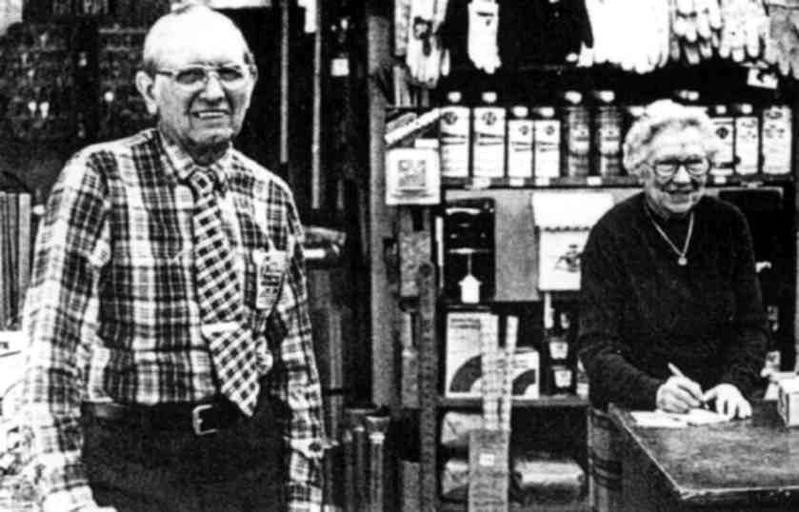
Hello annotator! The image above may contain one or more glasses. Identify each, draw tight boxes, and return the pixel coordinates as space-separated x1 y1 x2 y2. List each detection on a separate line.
639 155 714 179
148 60 254 85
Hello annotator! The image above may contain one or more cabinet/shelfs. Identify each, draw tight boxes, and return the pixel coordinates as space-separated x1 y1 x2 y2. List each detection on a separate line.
369 0 799 512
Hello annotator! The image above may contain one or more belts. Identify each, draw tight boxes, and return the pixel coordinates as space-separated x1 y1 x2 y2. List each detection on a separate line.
79 399 259 438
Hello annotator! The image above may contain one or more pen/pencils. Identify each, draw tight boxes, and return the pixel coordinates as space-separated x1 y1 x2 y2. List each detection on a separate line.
667 363 709 411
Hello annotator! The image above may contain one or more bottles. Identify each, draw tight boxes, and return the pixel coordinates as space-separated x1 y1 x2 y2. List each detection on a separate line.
438 89 470 182
595 91 623 180
471 92 508 181
761 104 792 181
562 89 591 180
533 107 562 180
734 104 761 179
505 105 534 180
709 104 735 181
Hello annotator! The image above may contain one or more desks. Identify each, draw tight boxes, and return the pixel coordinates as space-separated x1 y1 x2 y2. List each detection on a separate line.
607 402 799 510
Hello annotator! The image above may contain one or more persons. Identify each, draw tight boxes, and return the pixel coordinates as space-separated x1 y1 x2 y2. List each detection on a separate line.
15 6 329 512
579 102 772 512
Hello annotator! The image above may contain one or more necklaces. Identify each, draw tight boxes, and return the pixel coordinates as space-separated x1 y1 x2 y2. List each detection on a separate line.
641 198 694 266
